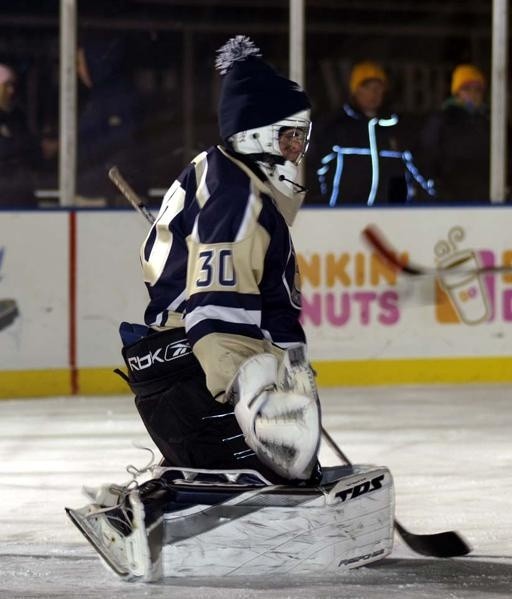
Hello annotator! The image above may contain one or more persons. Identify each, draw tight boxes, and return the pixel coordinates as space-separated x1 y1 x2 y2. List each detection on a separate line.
60 66 323 577
308 58 491 205
0 42 146 210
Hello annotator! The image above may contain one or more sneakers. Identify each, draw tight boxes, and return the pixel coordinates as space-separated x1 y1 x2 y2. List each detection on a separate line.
83 483 136 578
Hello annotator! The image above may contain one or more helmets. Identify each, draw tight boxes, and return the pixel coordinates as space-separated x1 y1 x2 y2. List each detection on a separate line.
229 103 313 160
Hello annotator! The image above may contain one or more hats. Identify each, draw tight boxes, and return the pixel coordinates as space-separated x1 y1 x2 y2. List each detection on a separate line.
349 62 387 91
212 34 312 137
451 62 483 95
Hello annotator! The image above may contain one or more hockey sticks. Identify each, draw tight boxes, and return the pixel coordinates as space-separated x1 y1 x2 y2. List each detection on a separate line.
108 166 470 558
